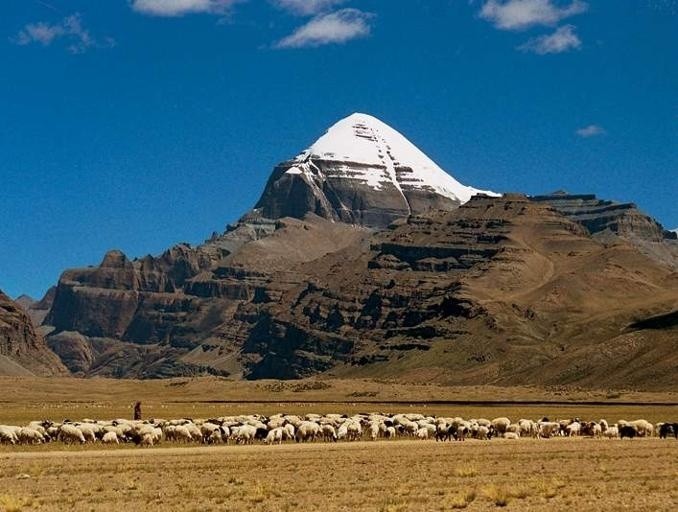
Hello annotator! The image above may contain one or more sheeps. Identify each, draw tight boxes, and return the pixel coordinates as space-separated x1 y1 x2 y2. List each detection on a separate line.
0 411 678 448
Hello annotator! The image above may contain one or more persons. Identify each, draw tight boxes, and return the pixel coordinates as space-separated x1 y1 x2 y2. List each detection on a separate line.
133 402 142 420
